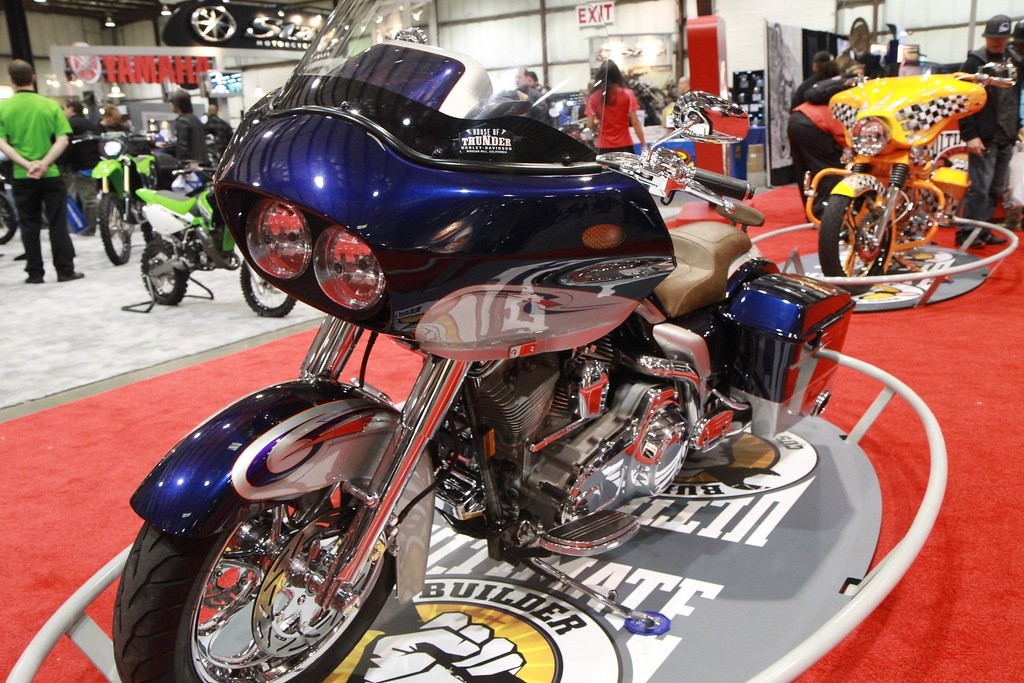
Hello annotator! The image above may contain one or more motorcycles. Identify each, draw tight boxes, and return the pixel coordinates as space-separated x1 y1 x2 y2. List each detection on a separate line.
110 1 857 681
70 131 159 268
804 73 998 292
132 159 299 320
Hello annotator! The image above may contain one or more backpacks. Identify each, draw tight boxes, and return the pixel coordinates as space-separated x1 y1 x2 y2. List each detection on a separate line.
805 73 859 106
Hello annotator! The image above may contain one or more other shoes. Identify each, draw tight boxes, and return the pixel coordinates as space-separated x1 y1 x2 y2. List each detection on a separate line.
25 275 44 283
956 231 982 247
980 230 1007 243
57 271 85 283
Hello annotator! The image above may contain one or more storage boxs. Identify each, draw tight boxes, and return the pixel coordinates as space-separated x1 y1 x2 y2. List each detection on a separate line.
728 272 855 442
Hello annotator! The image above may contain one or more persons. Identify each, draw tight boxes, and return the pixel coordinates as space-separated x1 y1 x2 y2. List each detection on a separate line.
64 101 96 134
955 14 1024 248
0 59 85 283
516 68 550 123
206 105 232 141
678 76 690 95
96 107 129 135
585 60 647 153
792 51 838 106
789 102 845 224
169 91 209 163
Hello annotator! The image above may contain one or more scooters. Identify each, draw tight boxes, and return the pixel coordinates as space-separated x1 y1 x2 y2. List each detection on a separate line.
0 173 20 246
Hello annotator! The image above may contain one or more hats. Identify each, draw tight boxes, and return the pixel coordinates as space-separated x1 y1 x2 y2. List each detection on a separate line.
171 91 194 114
982 15 1011 39
1012 21 1024 39
